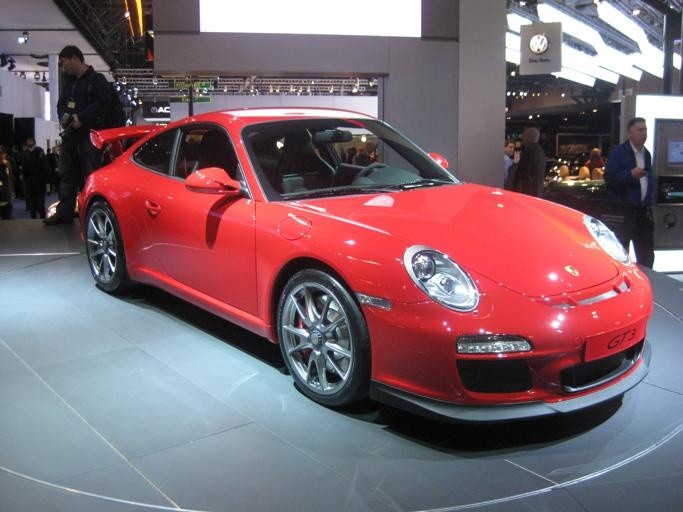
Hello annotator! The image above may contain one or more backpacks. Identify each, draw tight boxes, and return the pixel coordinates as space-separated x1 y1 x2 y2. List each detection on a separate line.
87 72 122 128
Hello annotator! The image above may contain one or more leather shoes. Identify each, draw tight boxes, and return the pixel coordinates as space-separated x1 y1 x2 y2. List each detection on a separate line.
43 213 63 225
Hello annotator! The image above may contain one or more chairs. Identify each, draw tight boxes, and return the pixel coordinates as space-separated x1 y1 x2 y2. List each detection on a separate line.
199 127 337 197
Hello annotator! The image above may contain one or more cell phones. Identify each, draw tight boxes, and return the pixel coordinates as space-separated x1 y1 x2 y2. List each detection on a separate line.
643 166 649 171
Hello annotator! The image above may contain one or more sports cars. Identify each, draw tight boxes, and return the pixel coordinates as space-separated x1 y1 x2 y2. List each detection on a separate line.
71 106 655 426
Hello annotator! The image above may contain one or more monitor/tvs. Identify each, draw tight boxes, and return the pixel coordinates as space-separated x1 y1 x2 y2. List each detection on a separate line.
667 140 683 165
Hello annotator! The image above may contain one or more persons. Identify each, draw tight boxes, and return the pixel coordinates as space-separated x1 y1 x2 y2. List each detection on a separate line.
602 118 655 268
503 140 516 184
0 137 58 219
515 127 547 200
42 47 112 225
341 140 378 168
584 148 603 177
